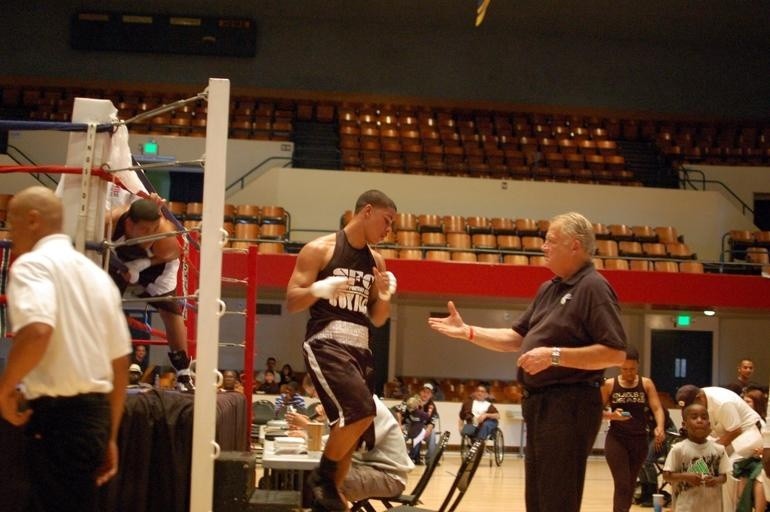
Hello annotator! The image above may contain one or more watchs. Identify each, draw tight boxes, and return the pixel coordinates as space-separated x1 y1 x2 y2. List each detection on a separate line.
551 346 561 366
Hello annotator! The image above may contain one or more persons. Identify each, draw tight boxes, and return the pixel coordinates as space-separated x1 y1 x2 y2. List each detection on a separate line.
727 360 755 395
131 343 152 383
285 372 415 512
275 382 307 418
458 386 500 456
257 357 281 383
401 398 433 465
153 365 179 392
1 185 132 509
663 405 730 512
105 199 191 393
740 390 768 427
599 347 665 511
738 438 770 512
634 406 674 507
217 370 244 395
288 188 398 512
126 364 154 394
281 363 299 386
676 386 765 512
259 370 280 395
398 384 441 467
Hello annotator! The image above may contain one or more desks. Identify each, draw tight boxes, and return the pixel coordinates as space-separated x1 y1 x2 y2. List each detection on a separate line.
263 441 324 508
259 425 307 444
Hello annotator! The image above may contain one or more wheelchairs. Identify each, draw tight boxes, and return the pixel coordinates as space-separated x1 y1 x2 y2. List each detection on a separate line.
398 407 505 466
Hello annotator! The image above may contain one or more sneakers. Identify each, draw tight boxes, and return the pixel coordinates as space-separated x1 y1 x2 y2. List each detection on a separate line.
306 469 347 512
167 349 195 392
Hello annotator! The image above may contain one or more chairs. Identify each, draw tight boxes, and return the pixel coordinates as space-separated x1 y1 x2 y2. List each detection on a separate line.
382 376 522 403
350 431 450 512
658 392 675 409
0 194 291 254
383 439 485 512
719 230 770 273
654 459 672 493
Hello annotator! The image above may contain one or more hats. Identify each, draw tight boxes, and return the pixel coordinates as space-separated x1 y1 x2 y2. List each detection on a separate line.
423 383 434 390
675 384 700 417
129 363 143 373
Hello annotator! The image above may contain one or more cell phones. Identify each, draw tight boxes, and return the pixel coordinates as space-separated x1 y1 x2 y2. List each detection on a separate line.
621 412 632 418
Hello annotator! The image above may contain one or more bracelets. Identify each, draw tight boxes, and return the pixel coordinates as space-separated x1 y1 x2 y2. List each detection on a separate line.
429 214 629 512
469 324 473 342
608 412 611 419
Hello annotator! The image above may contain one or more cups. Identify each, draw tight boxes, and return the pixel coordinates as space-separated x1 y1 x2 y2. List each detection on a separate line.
653 494 664 512
308 423 323 452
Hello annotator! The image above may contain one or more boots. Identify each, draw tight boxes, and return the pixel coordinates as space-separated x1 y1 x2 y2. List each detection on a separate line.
635 483 648 505
642 483 657 507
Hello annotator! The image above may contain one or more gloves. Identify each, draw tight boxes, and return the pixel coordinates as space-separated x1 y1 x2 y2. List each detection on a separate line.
116 257 152 285
385 271 397 295
309 275 348 299
